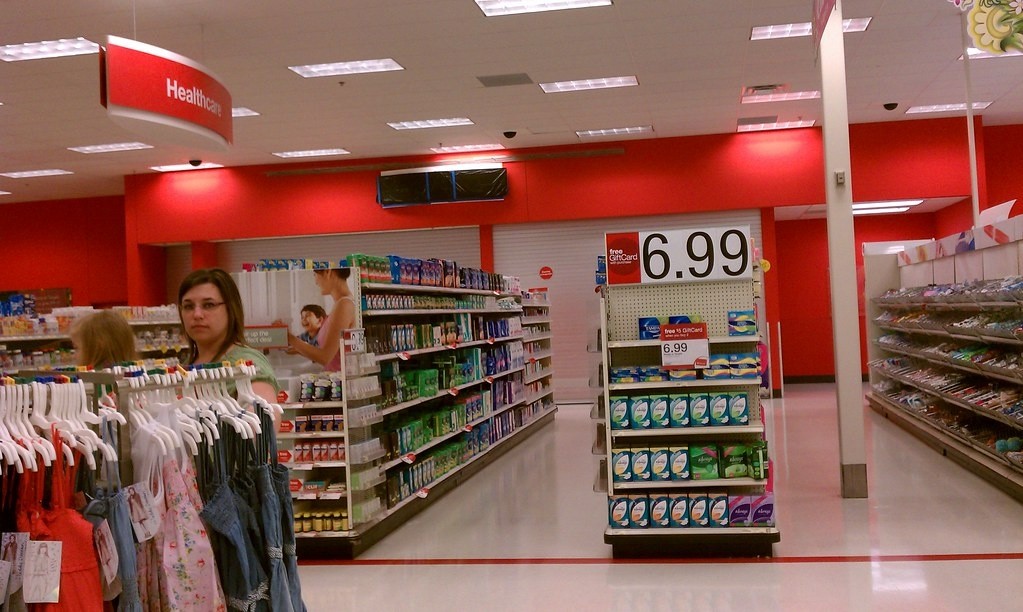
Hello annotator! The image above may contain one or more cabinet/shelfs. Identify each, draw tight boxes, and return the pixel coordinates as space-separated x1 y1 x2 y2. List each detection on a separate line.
864 214 1023 505
600 279 781 558
230 267 558 560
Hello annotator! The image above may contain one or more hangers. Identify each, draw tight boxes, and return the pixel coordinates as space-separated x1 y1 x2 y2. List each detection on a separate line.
0 365 283 476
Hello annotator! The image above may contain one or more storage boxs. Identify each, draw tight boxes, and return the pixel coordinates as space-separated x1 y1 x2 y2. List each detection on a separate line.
610 391 749 430
0 294 38 319
608 490 776 529
638 314 704 340
610 352 762 380
595 256 607 284
726 310 755 336
0 320 197 376
612 441 768 480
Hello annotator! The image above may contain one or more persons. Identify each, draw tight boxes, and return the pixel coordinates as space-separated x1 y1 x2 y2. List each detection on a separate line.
287 304 328 354
73 310 136 370
272 268 356 372
178 269 281 437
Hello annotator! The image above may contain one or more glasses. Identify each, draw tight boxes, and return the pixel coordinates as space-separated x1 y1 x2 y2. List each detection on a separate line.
179 300 227 312
